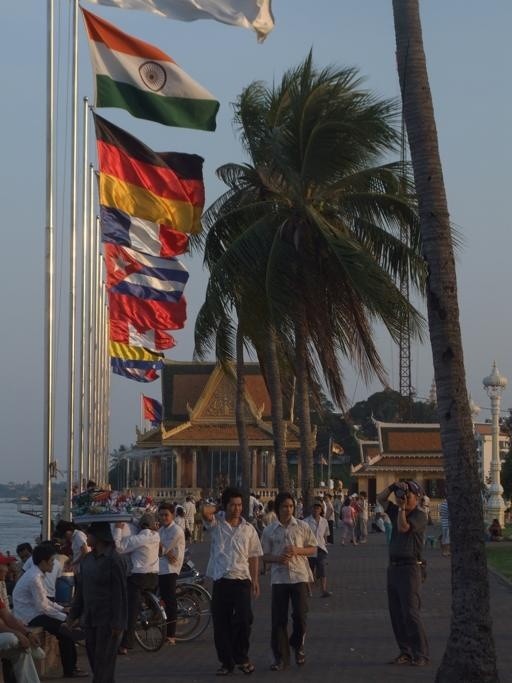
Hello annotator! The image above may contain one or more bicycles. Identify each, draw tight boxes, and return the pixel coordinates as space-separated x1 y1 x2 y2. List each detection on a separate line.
124 580 216 651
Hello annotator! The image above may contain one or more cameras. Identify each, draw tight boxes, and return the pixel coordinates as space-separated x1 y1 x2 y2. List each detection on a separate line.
395 488 411 500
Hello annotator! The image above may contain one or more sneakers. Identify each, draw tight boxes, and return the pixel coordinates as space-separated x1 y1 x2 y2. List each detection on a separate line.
341 540 367 546
392 655 410 665
28 644 46 660
410 657 432 667
63 668 89 677
59 622 86 641
162 636 176 646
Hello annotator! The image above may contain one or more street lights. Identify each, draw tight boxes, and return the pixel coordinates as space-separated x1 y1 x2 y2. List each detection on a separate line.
478 358 509 530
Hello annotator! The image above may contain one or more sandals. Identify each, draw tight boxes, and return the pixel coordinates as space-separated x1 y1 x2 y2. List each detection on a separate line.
215 663 235 676
239 663 255 674
289 637 305 665
271 663 288 670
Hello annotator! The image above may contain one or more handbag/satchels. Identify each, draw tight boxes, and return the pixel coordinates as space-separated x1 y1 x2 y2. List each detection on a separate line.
55 570 77 607
184 528 191 540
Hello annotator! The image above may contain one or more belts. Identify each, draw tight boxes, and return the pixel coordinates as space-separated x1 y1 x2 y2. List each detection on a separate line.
390 561 423 566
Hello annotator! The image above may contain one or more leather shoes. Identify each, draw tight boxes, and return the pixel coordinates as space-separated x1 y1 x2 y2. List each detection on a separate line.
117 646 128 654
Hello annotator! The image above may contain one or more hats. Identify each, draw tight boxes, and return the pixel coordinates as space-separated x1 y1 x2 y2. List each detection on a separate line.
86 521 116 543
0 552 16 564
140 513 154 528
403 479 425 497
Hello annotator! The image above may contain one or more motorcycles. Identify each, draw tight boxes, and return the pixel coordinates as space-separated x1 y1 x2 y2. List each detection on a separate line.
174 552 205 583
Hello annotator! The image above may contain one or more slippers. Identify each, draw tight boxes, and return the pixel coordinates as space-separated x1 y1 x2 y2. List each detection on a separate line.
319 591 333 598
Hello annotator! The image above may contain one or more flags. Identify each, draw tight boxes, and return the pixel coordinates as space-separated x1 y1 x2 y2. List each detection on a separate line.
332 441 345 455
323 457 328 466
